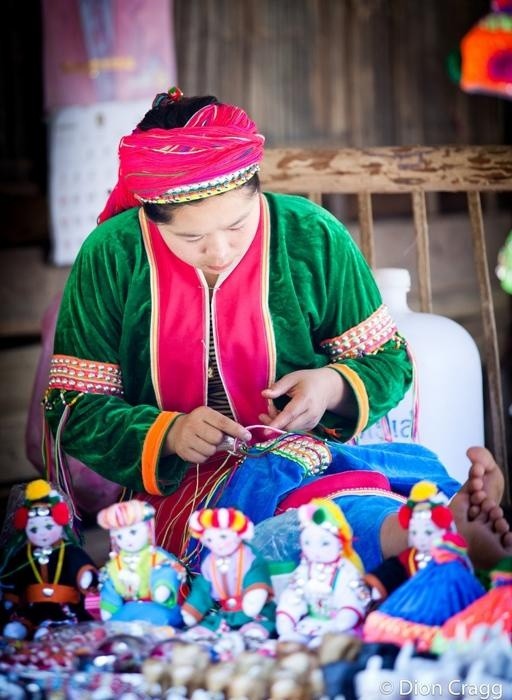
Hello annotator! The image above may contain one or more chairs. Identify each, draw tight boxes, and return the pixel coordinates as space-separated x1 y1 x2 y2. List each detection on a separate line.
255 144 512 521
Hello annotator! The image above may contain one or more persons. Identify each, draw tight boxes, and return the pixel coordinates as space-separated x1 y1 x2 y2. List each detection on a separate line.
96 500 185 638
430 558 512 664
0 479 100 642
40 88 512 580
274 496 372 648
361 484 482 661
181 508 274 645
